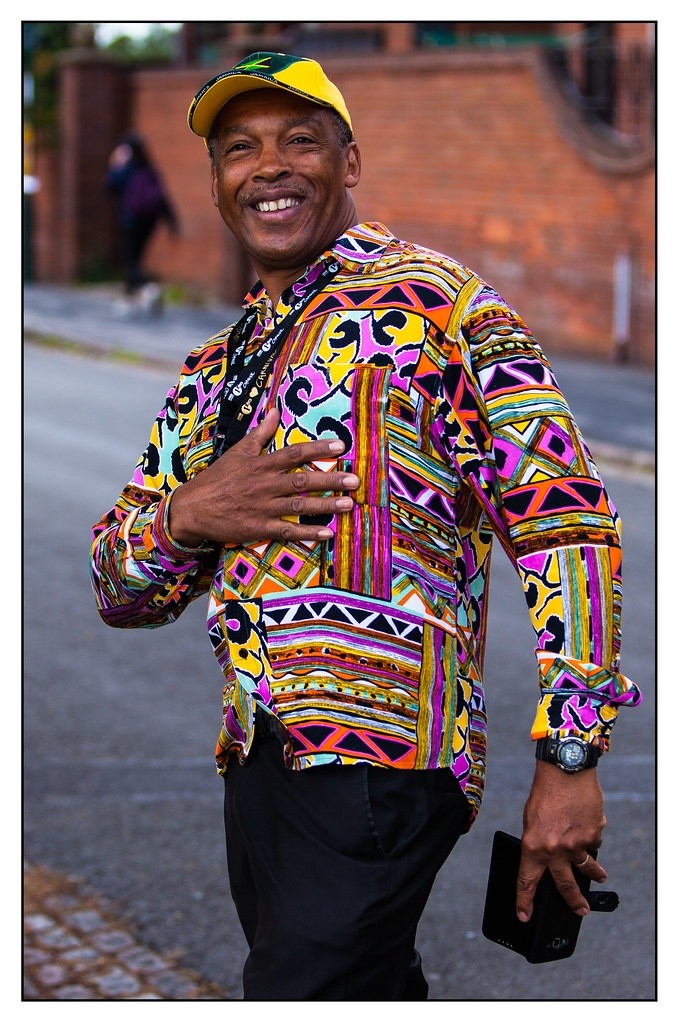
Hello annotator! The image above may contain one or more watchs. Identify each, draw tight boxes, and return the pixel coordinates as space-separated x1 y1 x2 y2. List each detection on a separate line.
533 734 604 774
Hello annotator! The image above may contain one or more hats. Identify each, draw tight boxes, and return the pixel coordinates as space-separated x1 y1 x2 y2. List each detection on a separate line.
186 50 351 137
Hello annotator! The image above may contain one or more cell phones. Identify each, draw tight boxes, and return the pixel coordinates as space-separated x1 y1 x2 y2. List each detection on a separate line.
527 848 599 964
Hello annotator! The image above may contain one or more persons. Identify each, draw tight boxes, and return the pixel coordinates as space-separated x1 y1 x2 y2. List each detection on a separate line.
85 50 644 1002
81 130 183 321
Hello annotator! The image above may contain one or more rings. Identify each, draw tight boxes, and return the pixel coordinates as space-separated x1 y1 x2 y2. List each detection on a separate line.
577 854 591 867
